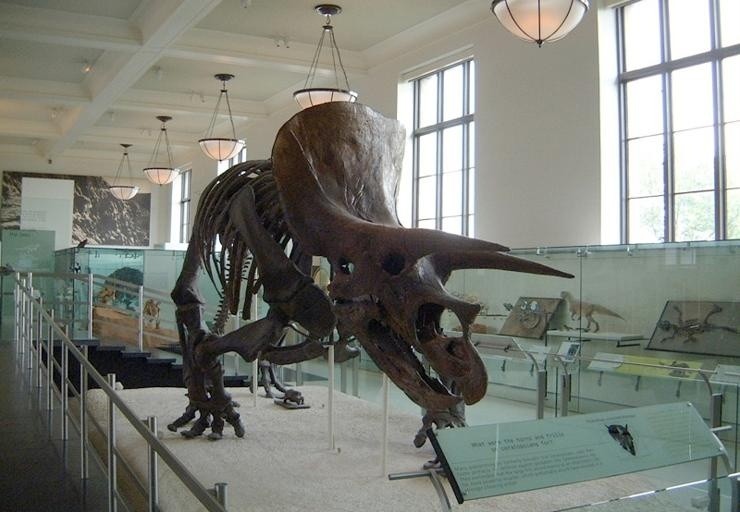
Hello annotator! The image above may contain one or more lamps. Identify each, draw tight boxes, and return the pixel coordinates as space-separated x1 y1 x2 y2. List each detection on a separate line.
489 0 590 52
197 73 246 163
108 143 140 203
142 115 181 188
294 3 359 111
